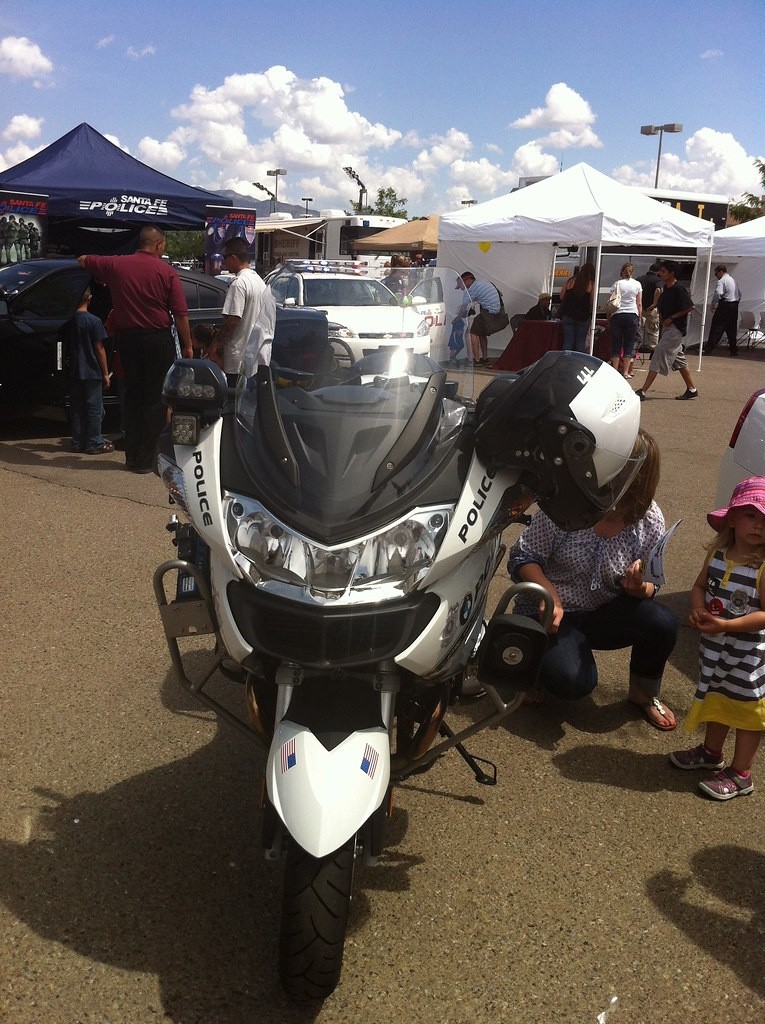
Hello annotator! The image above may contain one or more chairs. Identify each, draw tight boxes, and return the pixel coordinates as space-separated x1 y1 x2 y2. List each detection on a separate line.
717 312 765 355
510 315 525 333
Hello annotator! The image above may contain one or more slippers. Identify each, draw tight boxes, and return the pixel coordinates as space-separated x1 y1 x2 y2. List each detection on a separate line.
629 695 677 731
522 690 544 706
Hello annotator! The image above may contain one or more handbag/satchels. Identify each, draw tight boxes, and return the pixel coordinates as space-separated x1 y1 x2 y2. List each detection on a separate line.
480 306 509 336
605 281 621 318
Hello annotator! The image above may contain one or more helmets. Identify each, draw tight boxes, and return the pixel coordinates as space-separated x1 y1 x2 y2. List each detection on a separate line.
474 351 641 531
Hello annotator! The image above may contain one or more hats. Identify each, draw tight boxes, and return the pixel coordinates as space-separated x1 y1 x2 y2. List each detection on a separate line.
707 475 765 533
455 271 474 289
1 215 34 226
650 263 661 272
539 293 552 300
455 304 475 315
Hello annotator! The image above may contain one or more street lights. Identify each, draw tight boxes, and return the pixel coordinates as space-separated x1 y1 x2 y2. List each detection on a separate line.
641 122 684 189
266 168 287 202
301 197 314 218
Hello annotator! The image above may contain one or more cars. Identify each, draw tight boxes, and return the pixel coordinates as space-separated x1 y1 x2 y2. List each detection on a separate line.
259 258 451 375
171 259 199 271
0 259 334 422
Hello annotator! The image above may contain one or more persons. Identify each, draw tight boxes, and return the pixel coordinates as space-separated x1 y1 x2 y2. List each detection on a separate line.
451 272 503 367
697 265 742 356
525 293 552 320
669 474 765 800
0 215 39 264
559 262 595 352
67 223 215 473
508 427 678 730
635 260 698 400
208 237 277 389
606 263 662 378
381 252 437 302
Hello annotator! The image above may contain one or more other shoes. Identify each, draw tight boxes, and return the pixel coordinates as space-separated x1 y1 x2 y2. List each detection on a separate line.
126 461 153 473
623 374 632 379
8 260 13 264
697 345 712 355
72 439 114 454
731 351 738 356
448 358 489 367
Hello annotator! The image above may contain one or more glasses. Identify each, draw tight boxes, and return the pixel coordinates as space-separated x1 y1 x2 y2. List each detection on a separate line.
223 253 237 261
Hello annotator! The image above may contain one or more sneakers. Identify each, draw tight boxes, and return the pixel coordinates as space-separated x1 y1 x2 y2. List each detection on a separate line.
635 389 647 401
675 388 698 399
670 744 724 770
698 767 754 800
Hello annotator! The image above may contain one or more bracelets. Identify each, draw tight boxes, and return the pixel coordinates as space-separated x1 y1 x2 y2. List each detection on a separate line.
670 316 673 320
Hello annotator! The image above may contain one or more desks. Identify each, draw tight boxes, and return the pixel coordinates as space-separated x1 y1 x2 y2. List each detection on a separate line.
492 319 623 371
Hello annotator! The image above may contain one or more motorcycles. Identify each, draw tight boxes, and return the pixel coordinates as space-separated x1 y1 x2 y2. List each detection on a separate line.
150 266 554 1012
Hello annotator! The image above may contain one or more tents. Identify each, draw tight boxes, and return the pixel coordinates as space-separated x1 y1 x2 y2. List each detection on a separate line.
351 215 440 261
438 161 716 372
685 216 765 350
0 123 233 232
